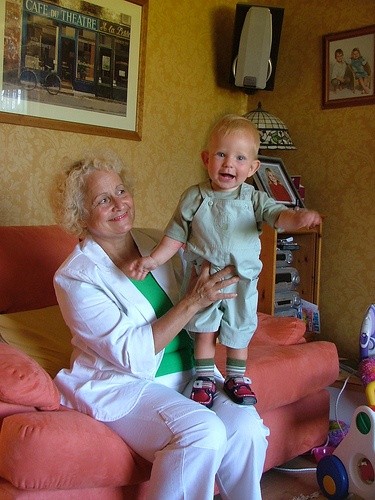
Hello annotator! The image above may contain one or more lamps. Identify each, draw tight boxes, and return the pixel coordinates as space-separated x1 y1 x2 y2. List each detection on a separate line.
240 102 296 157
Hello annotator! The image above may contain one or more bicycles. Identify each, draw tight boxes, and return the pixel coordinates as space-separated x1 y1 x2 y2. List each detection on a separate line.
16 59 62 95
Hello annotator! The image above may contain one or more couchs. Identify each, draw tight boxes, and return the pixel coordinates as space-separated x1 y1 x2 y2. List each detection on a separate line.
0 224 340 500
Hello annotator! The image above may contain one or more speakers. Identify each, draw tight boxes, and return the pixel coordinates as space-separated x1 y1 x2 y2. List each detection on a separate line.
230 4 284 92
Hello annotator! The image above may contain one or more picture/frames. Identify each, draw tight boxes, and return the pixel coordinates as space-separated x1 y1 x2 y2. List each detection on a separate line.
321 24 375 110
251 156 307 211
0 0 148 141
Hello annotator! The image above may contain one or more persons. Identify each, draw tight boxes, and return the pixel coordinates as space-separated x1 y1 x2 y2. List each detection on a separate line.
51 154 271 500
263 165 293 202
128 115 322 410
329 48 373 94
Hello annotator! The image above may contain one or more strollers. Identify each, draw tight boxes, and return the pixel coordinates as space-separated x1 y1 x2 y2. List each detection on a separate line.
316 303 374 500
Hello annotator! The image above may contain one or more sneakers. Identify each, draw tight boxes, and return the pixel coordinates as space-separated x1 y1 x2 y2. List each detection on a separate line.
189 377 216 407
223 376 257 404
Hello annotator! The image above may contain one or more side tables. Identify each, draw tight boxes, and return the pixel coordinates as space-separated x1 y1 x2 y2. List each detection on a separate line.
258 209 328 343
